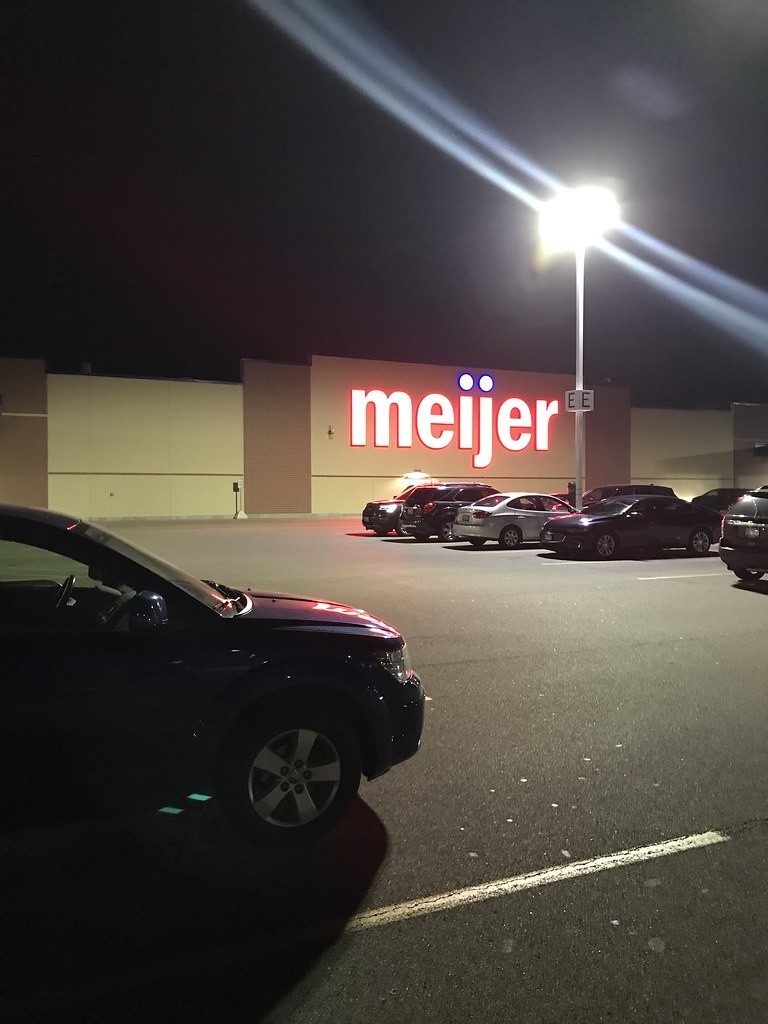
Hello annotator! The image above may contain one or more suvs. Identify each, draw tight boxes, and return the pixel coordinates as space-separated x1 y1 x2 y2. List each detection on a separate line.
362 482 491 536
719 485 768 582
582 485 678 499
400 486 499 542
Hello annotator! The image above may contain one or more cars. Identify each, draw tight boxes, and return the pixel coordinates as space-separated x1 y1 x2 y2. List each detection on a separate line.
539 494 722 561
1 503 425 838
453 492 580 548
691 488 756 516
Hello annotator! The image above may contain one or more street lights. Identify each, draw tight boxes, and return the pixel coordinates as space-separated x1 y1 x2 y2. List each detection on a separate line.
540 187 621 505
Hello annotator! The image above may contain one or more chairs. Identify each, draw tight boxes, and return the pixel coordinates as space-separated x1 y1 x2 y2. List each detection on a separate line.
515 499 521 509
527 504 534 510
